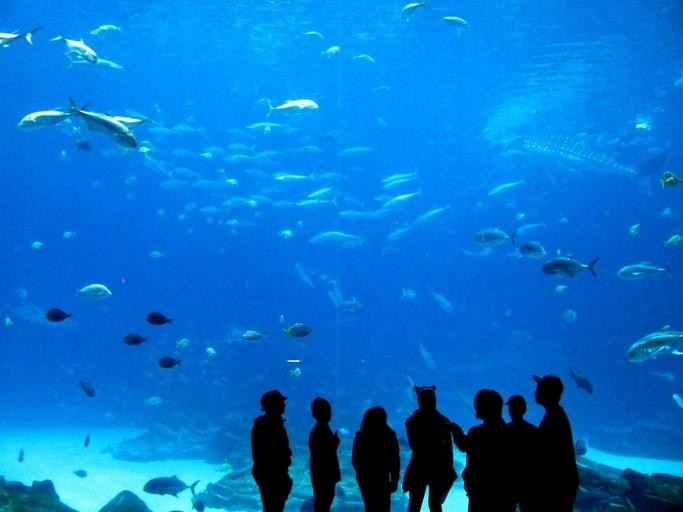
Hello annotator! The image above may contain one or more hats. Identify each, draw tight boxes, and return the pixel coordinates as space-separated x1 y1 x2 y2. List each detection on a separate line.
260 389 287 411
532 374 564 396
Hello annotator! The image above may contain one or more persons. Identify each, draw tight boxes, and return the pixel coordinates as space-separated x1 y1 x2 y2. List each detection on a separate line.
503 397 540 511
307 397 341 511
444 388 508 511
251 390 293 512
351 405 399 511
402 383 458 511
530 373 580 510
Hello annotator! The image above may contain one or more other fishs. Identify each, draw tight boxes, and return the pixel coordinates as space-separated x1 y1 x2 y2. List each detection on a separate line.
1 0 683 456
